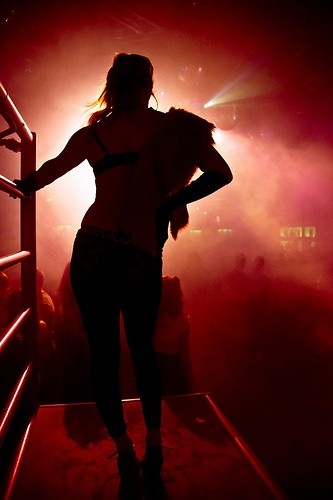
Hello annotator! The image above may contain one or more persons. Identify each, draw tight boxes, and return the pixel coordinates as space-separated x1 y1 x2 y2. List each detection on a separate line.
154 276 193 394
184 252 271 299
1 262 93 404
7 53 233 483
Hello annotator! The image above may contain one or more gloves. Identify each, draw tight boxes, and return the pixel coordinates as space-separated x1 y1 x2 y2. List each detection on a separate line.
9 170 40 198
155 168 230 250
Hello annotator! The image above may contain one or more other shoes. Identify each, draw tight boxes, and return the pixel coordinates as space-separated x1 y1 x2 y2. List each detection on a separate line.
138 445 163 483
114 449 140 484
